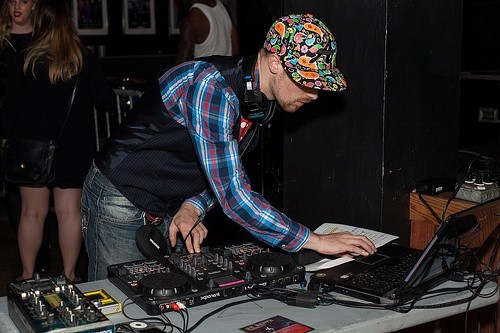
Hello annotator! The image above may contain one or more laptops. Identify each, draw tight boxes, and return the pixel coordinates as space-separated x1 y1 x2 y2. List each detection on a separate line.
313 197 500 308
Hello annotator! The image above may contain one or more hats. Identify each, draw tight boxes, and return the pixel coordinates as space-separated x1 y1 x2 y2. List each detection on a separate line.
264 14 347 91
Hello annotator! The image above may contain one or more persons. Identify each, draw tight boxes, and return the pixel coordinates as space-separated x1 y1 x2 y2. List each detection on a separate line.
0 0 117 284
176 0 241 65
79 15 377 282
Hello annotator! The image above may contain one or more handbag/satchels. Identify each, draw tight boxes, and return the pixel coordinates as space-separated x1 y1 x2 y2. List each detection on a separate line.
2 136 58 187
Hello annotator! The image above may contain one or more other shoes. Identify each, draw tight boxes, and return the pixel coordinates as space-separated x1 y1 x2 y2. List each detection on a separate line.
15 275 25 281
71 277 83 284
